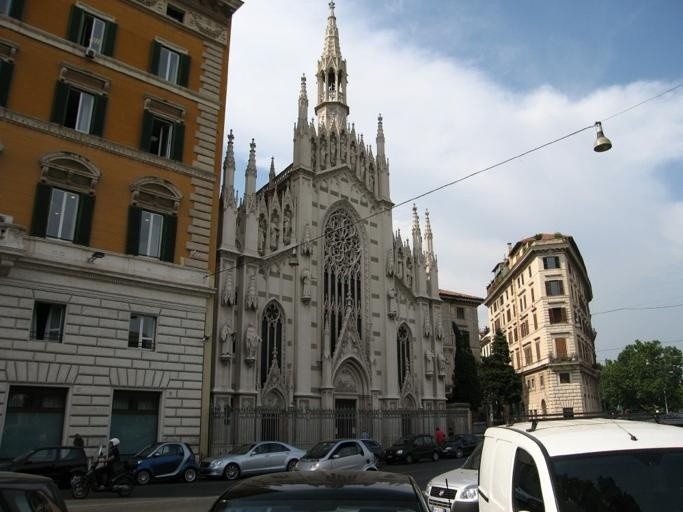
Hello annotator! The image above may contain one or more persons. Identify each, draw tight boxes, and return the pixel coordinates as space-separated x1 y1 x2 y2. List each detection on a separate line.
447 427 454 441
624 406 631 419
435 427 445 447
97 436 121 490
73 432 84 448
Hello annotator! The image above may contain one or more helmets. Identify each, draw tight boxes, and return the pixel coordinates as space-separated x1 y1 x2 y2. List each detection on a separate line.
106 437 126 451
